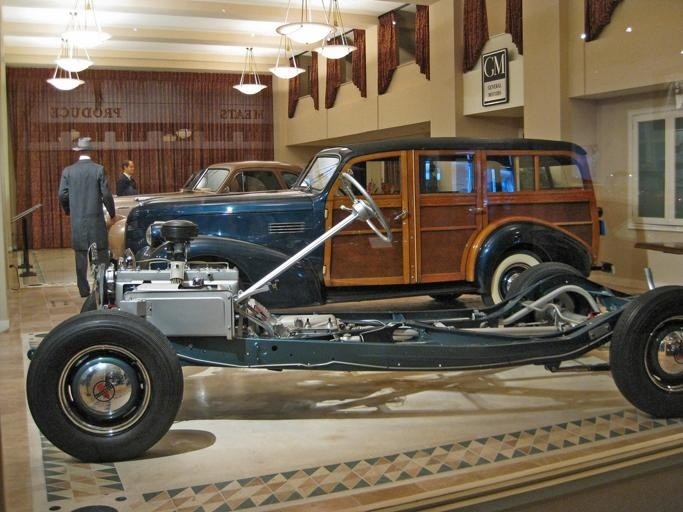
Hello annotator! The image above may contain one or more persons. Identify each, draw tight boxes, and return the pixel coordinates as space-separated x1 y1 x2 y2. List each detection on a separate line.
117 160 137 196
57 137 115 296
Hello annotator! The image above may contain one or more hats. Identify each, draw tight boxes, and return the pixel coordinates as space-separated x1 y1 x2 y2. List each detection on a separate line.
72 136 98 151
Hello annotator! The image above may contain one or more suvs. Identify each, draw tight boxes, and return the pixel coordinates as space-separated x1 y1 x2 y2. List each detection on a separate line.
120 127 618 319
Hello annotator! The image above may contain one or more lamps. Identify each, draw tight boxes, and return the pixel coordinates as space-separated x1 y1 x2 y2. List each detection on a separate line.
233 0 359 95
46 0 112 90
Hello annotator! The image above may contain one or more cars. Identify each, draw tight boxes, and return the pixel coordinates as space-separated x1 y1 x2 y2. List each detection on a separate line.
87 159 313 273
